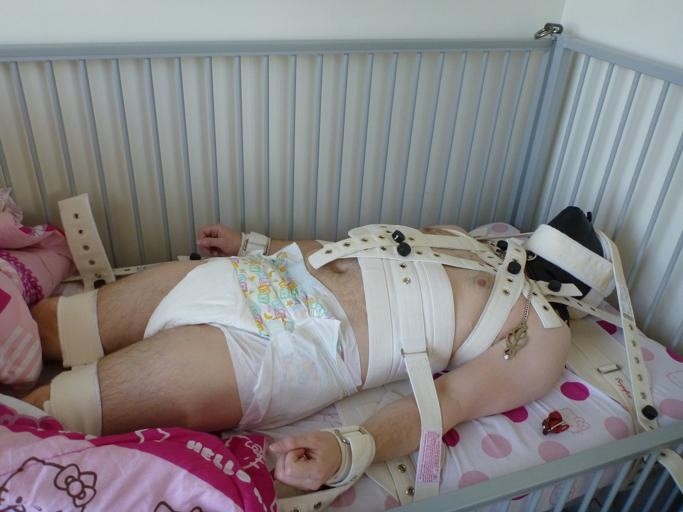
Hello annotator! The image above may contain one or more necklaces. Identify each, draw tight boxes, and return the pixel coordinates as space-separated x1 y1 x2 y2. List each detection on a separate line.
505 285 533 359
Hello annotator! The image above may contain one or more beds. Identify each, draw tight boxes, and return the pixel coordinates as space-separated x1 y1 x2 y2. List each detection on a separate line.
0 27 682 512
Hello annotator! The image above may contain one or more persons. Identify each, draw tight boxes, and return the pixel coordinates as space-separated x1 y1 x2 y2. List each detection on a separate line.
20 206 616 493
